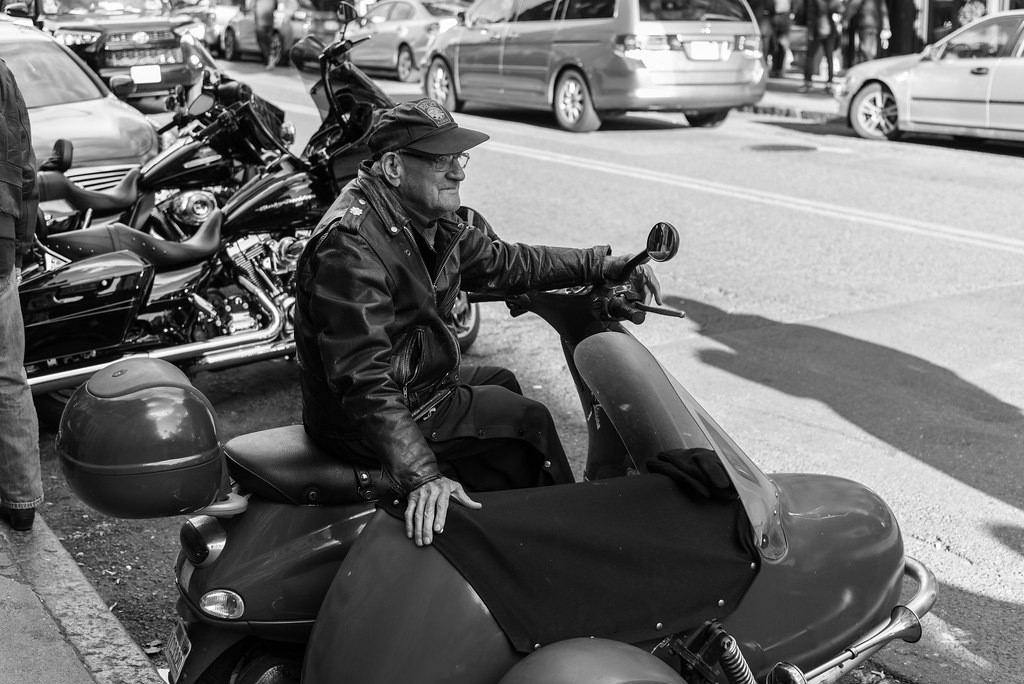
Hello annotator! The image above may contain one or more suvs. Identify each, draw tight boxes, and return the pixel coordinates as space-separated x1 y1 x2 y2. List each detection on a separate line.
5 0 203 108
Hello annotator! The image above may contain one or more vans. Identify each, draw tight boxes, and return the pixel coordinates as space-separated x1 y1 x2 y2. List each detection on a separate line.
419 0 765 132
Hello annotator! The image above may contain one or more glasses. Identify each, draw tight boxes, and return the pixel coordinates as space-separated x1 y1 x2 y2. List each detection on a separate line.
400 150 473 171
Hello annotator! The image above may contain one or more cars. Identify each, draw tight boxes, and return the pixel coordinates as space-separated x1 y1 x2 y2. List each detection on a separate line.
835 10 1024 142
0 26 169 228
225 0 346 66
170 0 243 58
333 0 469 81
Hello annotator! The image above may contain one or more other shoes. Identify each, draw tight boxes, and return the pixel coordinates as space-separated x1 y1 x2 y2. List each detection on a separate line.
799 81 817 93
1 498 37 533
823 82 836 95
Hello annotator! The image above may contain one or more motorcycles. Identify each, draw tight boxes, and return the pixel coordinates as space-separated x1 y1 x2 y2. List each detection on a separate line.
31 72 301 240
51 206 941 684
17 34 482 440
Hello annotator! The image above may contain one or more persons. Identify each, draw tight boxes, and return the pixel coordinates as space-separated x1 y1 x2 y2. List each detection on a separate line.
294 98 661 547
0 57 47 530
766 0 879 94
246 0 280 70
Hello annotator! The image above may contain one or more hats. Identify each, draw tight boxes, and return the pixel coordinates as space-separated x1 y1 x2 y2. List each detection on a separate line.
366 97 490 160
